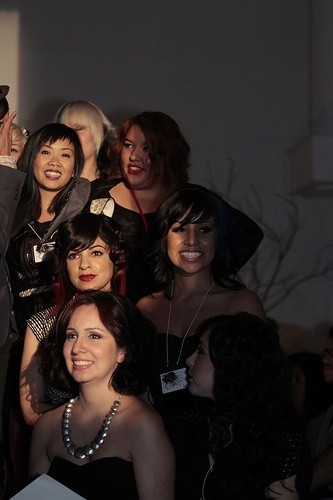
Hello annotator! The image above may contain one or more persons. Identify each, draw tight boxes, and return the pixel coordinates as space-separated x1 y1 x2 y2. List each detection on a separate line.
0 84 117 419
283 326 333 500
80 112 264 306
167 315 313 500
29 290 175 500
136 185 265 452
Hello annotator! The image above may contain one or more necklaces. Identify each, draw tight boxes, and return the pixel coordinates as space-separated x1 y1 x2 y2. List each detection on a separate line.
130 186 149 232
63 394 123 459
